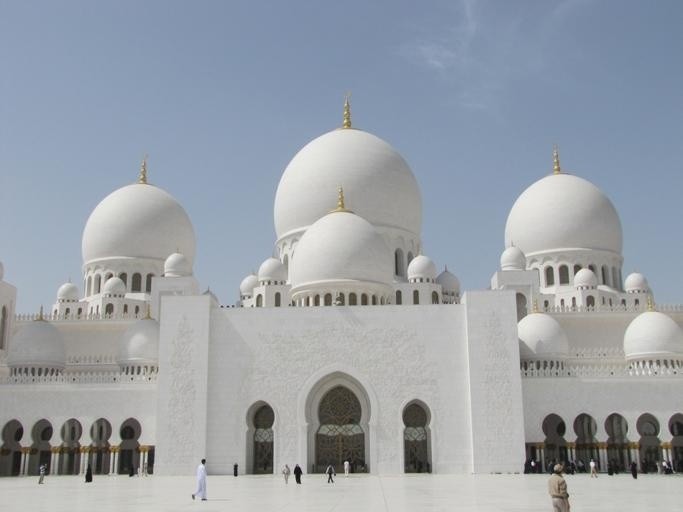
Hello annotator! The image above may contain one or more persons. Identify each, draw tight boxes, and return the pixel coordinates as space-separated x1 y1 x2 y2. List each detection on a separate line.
37 462 47 484
325 463 334 483
548 463 570 511
349 459 354 473
282 464 289 483
589 458 597 479
84 463 91 482
232 462 238 477
630 461 638 479
523 456 682 475
120 461 148 477
190 457 207 501
293 463 302 485
342 458 349 476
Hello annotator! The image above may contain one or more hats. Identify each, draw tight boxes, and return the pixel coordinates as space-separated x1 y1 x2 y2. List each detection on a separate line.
553 464 564 472
